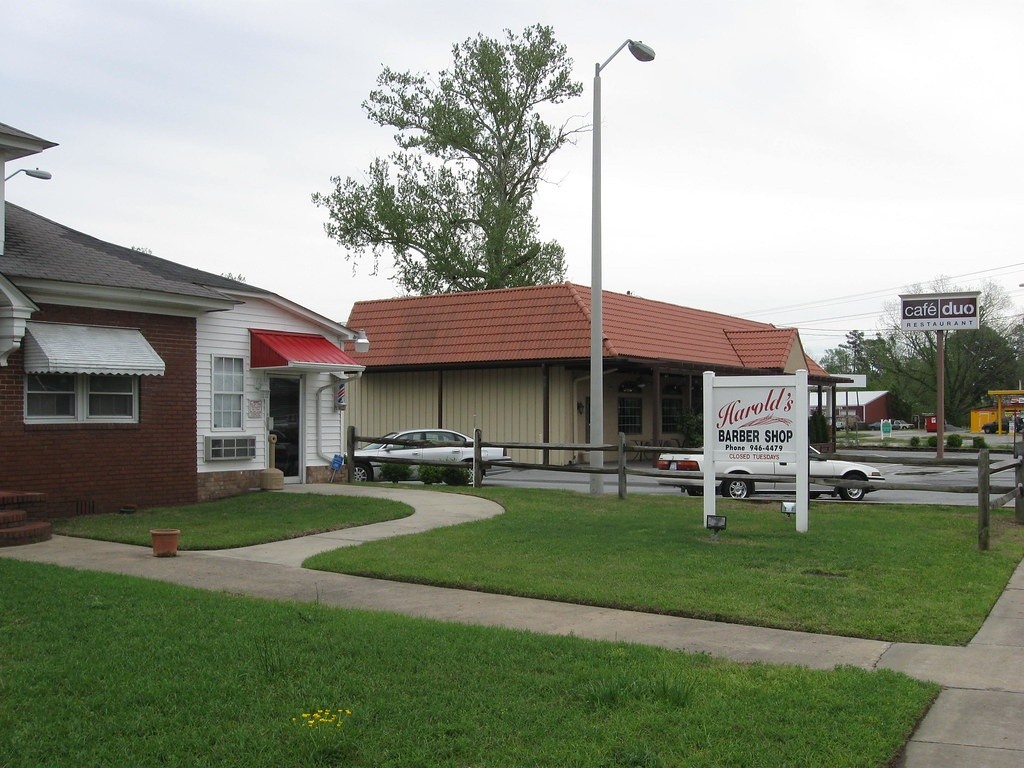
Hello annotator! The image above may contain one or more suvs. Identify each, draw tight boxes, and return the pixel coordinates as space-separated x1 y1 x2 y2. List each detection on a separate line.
981 417 1024 434
836 422 846 431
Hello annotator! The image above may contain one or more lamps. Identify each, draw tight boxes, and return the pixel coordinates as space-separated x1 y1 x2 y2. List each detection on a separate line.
577 402 585 415
706 514 727 533
779 501 796 517
4 168 52 181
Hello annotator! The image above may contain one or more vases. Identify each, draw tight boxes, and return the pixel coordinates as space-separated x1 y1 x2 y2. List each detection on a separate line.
149 528 181 557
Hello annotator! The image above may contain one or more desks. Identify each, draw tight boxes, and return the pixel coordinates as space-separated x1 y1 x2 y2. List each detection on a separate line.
668 436 694 456
630 438 655 461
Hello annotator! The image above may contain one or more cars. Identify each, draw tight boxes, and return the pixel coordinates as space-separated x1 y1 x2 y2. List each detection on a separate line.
868 420 916 430
352 429 511 487
654 443 887 502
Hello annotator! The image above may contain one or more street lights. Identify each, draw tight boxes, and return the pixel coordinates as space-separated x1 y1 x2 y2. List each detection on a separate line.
592 38 655 497
